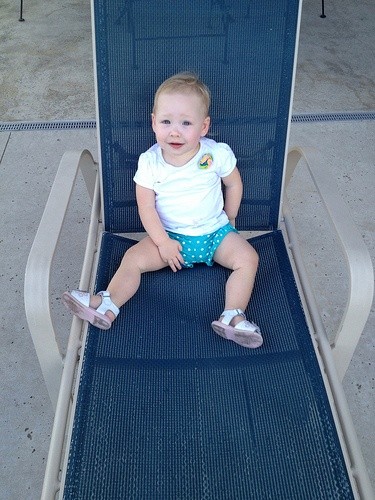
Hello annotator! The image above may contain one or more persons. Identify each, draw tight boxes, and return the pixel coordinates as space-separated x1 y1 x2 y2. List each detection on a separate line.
62 73 263 348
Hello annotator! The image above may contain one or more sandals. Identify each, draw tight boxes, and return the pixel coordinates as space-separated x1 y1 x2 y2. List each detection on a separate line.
211 308 263 349
61 289 120 330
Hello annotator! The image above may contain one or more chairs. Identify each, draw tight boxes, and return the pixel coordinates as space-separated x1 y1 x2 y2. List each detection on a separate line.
22 0 375 500
